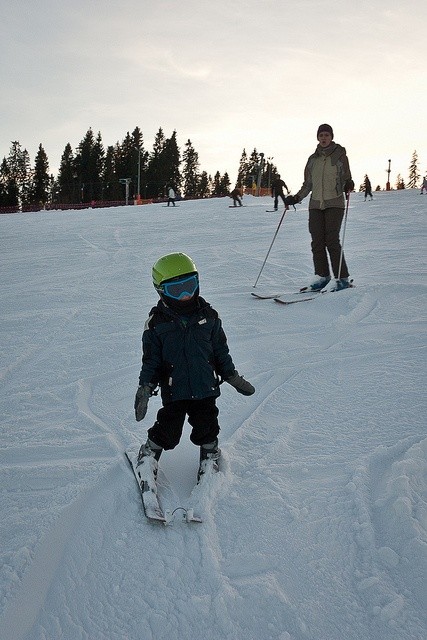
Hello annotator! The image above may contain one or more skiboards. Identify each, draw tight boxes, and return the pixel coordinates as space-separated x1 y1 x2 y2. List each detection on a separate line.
228 205 247 207
362 199 376 201
251 283 357 304
265 209 292 212
418 192 427 195
125 445 228 523
162 205 179 207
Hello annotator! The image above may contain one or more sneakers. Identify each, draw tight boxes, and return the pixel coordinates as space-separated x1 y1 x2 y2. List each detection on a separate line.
197 440 221 486
301 277 332 292
322 277 354 292
137 439 164 487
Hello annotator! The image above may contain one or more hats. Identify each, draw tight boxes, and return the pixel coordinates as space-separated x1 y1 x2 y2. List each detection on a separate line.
318 124 333 139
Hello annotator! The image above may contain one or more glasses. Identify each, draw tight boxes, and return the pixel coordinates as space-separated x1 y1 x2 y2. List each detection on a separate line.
161 275 199 300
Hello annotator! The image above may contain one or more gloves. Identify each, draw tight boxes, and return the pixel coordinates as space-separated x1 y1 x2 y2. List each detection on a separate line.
134 382 157 421
284 195 298 205
343 180 354 192
224 373 255 396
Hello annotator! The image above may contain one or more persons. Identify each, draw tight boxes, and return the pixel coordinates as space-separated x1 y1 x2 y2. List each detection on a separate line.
134 252 255 481
364 177 373 201
229 186 243 206
271 174 289 210
165 185 176 206
420 177 427 194
283 124 354 291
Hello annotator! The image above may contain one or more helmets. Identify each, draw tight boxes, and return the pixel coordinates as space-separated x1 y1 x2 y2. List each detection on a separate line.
152 253 199 304
